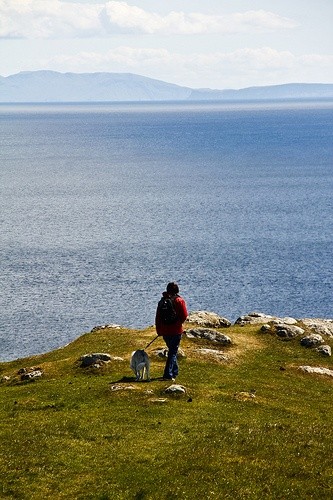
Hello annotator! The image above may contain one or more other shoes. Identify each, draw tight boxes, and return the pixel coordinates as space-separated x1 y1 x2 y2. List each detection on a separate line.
163 374 176 381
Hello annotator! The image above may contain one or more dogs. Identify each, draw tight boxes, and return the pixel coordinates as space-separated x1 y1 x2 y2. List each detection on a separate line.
130 349 150 382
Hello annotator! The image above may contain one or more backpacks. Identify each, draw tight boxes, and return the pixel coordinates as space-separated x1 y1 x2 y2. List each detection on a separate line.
156 295 180 323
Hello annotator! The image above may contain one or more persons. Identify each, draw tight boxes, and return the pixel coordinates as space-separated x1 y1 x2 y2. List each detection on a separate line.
154 282 187 381
